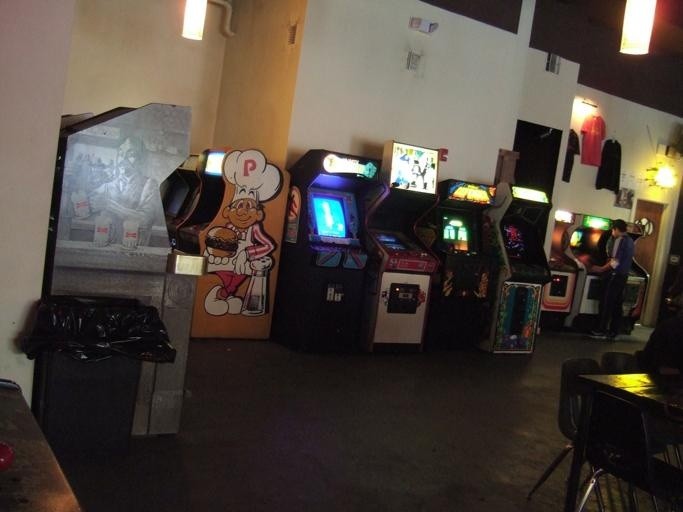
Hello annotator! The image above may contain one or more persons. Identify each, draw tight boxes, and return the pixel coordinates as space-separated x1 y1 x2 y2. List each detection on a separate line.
587 218 634 341
85 137 158 244
203 198 282 317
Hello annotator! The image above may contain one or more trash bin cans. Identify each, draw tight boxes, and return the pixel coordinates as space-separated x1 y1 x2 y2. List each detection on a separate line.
32 297 146 452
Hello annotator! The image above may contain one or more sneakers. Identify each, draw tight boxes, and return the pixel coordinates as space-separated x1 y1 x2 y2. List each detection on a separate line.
587 330 615 339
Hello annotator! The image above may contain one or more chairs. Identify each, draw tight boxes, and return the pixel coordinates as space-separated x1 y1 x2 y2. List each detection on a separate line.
522 348 681 510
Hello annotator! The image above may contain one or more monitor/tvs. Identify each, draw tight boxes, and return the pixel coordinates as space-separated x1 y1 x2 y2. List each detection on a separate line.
503 222 542 258
440 208 471 252
312 193 350 237
167 186 188 215
569 229 587 252
